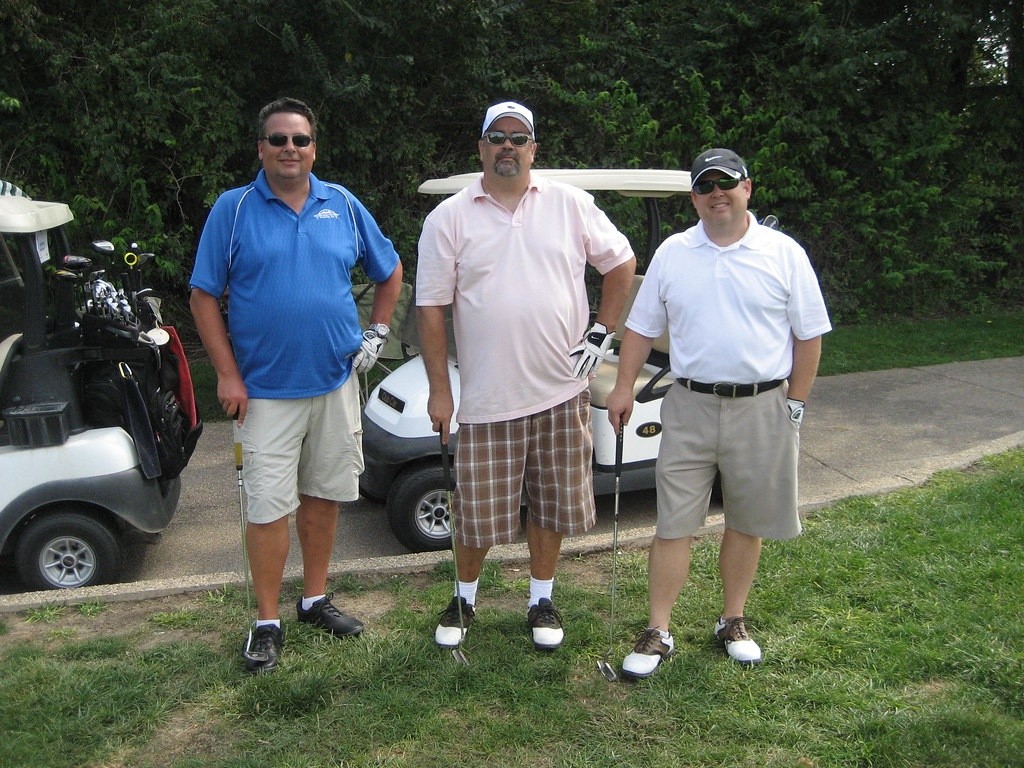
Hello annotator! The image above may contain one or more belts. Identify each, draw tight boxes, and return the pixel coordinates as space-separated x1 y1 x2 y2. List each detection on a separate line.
676 377 783 399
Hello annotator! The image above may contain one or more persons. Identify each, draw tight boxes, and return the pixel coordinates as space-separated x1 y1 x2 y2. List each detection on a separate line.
607 148 832 679
189 97 403 673
415 101 636 650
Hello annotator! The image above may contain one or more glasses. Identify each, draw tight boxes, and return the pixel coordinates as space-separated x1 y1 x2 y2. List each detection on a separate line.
482 131 532 146
692 176 746 194
261 133 314 147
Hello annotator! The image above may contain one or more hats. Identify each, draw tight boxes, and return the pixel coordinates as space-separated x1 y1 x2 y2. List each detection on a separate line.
691 148 748 188
482 102 535 145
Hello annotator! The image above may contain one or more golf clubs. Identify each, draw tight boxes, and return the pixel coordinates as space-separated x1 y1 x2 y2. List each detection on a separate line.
229 407 269 661
438 426 471 666
595 412 629 683
84 269 153 326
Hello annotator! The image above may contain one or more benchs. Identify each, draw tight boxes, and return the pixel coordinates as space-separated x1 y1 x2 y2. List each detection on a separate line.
588 275 675 407
0 334 23 391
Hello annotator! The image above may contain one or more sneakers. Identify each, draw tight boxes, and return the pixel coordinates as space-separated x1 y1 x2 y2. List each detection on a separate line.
525 598 565 650
296 591 364 638
435 595 477 648
240 624 284 672
622 625 675 678
713 616 761 665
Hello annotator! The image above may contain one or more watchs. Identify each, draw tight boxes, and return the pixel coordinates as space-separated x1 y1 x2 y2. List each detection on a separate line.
369 323 390 339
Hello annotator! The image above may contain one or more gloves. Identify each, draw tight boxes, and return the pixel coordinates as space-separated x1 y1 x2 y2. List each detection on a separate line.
568 322 616 382
788 398 804 426
351 330 389 375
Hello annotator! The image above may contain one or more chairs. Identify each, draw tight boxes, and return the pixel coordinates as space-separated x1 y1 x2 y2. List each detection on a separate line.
351 282 421 405
